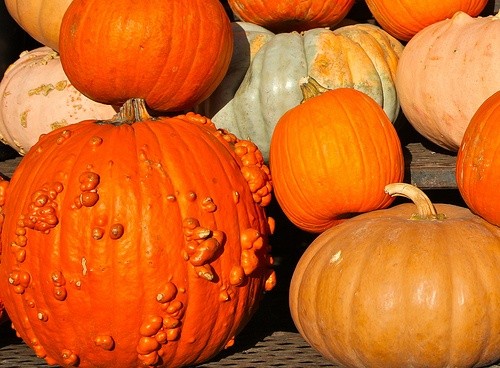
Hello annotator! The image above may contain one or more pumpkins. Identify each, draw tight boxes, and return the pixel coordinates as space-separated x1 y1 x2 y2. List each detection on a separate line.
0 0 500 368
